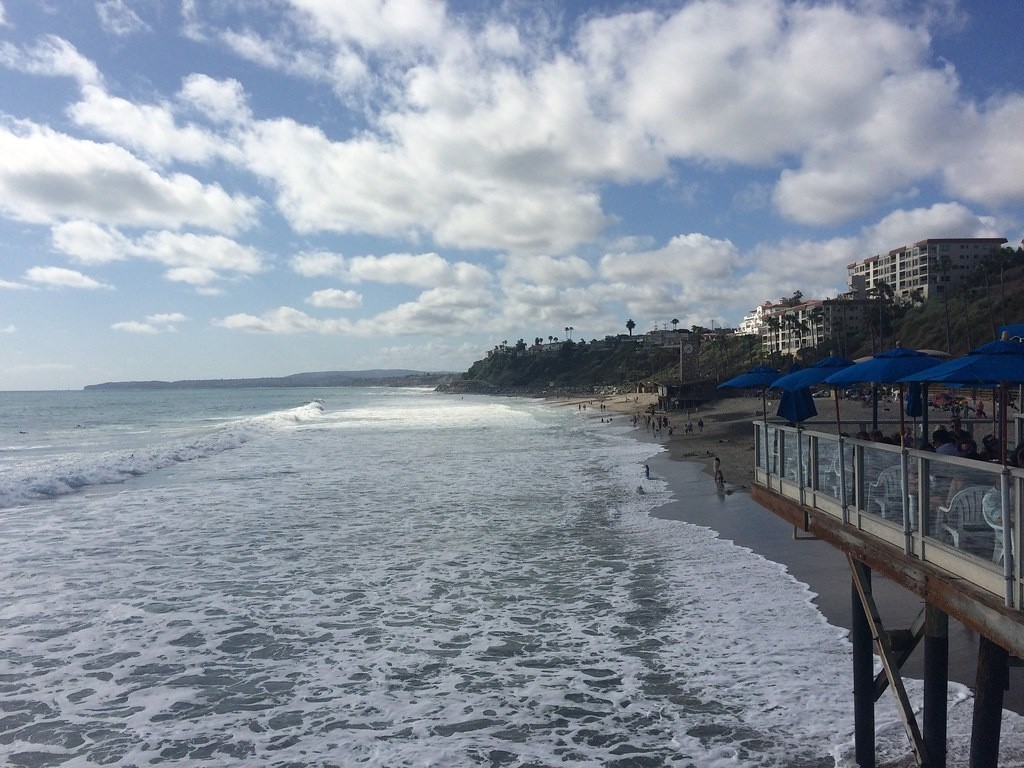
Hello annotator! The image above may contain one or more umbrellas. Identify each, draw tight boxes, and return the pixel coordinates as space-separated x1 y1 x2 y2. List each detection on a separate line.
715 330 1024 449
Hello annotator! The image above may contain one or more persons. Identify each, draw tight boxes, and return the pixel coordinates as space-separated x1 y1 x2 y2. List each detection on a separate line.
832 400 1024 565
645 464 650 479
601 417 613 424
631 406 704 436
718 469 724 482
577 400 606 412
713 457 720 480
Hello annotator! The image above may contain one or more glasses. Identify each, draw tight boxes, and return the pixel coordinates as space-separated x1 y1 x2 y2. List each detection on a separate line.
951 425 959 428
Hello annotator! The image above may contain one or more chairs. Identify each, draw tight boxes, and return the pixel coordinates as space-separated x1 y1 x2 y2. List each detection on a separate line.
771 445 1017 570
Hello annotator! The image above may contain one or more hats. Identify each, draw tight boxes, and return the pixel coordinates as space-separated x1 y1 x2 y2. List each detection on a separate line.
982 434 998 447
